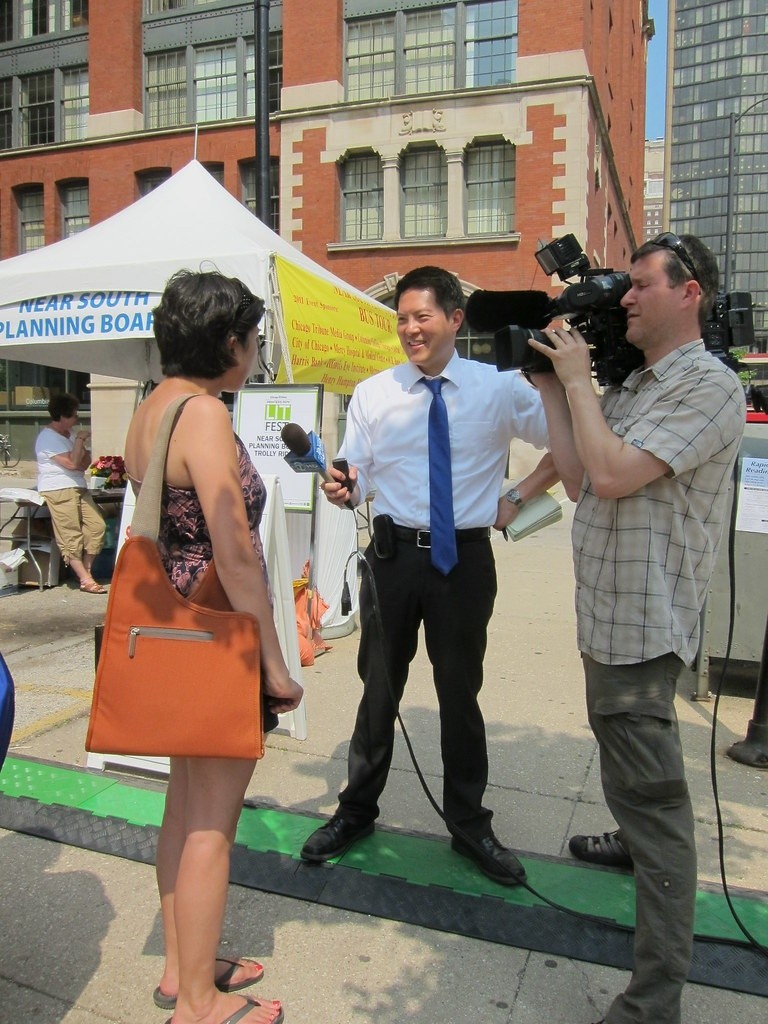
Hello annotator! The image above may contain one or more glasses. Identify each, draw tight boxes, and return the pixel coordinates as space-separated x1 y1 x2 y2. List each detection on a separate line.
238 330 266 348
68 412 80 419
653 232 704 295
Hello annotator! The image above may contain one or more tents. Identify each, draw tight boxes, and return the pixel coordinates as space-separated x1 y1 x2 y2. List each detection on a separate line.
0 160 457 622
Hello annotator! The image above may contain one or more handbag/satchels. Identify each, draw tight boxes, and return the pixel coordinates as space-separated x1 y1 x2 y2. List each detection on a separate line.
84 539 264 759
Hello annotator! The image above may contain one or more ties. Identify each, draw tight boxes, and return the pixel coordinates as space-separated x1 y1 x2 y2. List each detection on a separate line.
419 376 459 576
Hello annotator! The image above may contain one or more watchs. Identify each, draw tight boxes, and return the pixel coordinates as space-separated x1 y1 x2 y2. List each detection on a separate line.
507 489 525 509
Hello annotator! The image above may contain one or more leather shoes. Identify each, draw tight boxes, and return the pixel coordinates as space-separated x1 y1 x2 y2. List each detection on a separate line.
301 811 377 860
451 828 528 884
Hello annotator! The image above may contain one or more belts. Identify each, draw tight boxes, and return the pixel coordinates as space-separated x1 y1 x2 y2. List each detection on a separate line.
394 524 491 546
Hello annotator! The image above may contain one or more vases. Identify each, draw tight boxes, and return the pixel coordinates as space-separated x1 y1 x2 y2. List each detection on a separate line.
90 477 107 488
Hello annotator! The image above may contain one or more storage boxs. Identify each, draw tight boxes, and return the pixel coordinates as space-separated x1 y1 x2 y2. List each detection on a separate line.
12 517 59 587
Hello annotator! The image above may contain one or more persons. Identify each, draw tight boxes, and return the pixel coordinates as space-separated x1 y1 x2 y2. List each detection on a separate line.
34 393 108 594
529 232 742 1024
0 652 16 774
126 265 304 1023
296 266 562 886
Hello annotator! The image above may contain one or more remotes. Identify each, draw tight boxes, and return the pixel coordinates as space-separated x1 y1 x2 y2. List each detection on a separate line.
332 458 353 494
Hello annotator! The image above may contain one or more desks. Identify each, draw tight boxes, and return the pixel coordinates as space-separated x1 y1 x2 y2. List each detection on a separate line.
0 489 124 592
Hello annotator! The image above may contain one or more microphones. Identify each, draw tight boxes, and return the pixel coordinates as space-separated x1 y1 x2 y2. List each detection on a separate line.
465 289 563 334
280 423 354 513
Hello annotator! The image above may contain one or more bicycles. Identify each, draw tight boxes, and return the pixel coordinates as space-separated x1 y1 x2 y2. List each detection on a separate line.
0 434 21 468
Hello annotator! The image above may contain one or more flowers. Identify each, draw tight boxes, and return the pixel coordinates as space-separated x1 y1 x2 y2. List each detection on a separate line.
90 456 129 487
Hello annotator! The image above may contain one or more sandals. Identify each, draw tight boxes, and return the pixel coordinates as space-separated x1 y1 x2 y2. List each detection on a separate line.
570 830 636 871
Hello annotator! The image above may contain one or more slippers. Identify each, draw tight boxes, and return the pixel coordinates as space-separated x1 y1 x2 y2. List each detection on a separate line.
165 994 285 1024
79 581 108 594
153 957 264 1009
